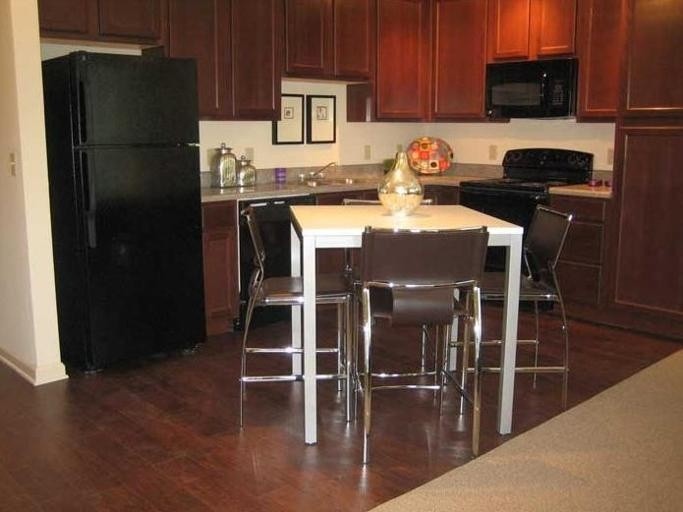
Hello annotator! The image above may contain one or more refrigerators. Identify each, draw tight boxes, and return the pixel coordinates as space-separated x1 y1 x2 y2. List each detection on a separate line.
43 50 211 372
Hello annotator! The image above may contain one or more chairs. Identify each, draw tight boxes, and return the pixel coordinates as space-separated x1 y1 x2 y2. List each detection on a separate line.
342 198 432 265
353 226 490 457
237 204 352 424
443 202 575 404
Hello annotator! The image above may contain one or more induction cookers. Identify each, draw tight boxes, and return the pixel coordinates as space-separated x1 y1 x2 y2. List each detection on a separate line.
472 171 569 191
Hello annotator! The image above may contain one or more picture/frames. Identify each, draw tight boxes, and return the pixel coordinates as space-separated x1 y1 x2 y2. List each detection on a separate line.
271 93 304 145
305 95 336 144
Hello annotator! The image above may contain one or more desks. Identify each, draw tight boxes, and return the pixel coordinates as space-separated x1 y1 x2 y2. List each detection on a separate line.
291 203 524 446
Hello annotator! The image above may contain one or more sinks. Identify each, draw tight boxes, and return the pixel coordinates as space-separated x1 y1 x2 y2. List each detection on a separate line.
300 179 328 188
332 177 364 185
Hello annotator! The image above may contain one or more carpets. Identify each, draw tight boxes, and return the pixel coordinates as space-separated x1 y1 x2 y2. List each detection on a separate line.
366 348 683 511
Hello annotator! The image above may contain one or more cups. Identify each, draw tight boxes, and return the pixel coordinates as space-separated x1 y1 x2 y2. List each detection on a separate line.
604 179 612 187
586 178 599 187
297 173 305 185
275 168 285 183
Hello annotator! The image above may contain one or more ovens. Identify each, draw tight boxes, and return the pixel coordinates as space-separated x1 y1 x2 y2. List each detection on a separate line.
458 184 549 310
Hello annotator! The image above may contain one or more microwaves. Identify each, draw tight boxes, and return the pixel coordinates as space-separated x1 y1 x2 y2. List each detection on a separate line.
484 59 576 119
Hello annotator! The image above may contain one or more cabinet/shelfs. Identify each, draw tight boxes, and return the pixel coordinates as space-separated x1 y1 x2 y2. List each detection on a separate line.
38 0 164 44
167 1 281 121
578 0 624 116
488 0 576 60
376 1 487 118
286 0 375 80
550 196 606 305
201 202 239 334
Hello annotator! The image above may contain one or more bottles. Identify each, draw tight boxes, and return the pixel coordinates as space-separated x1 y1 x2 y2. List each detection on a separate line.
215 144 255 188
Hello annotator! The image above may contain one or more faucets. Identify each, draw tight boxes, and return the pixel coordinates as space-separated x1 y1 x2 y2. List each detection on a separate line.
312 161 338 177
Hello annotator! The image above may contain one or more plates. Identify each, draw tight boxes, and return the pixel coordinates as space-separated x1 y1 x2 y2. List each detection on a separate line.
405 136 454 175
